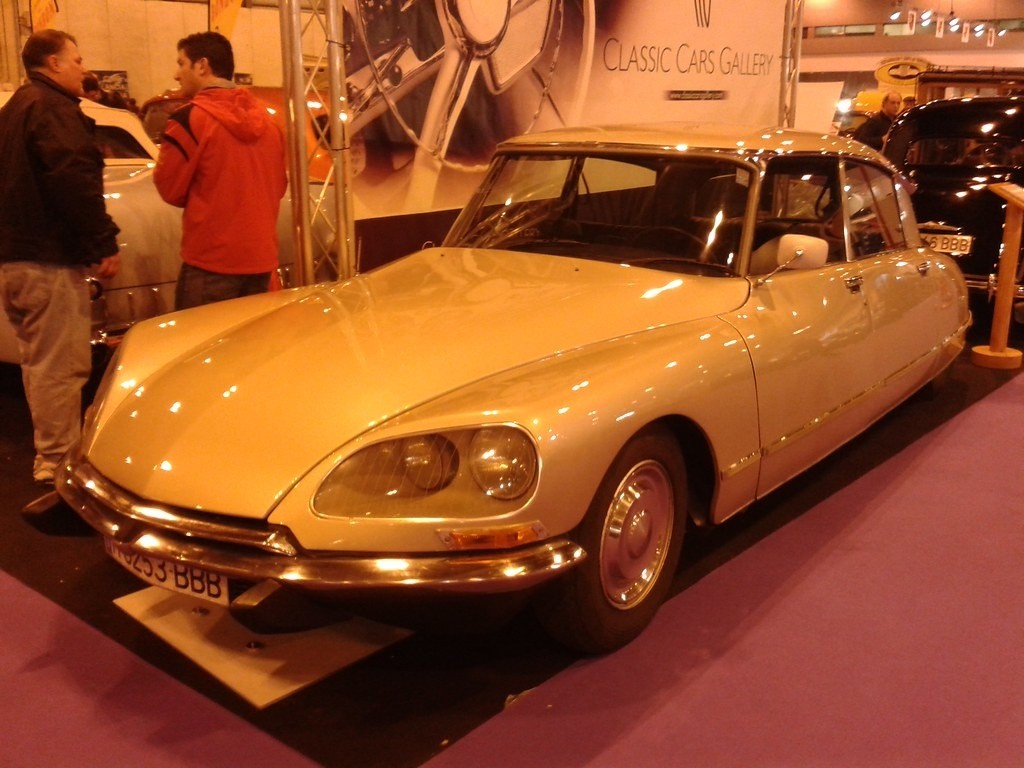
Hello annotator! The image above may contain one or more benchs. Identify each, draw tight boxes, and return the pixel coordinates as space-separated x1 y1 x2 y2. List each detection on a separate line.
688 215 856 273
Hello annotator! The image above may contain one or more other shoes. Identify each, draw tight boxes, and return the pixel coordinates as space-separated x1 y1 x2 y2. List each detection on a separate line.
33 455 64 485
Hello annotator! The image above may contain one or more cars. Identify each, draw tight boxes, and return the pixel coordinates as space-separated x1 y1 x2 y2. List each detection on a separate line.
877 90 1024 330
0 85 358 402
52 121 974 655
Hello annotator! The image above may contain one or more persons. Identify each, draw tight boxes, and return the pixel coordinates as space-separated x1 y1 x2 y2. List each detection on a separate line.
853 92 916 154
0 29 120 488
156 32 288 313
80 75 128 110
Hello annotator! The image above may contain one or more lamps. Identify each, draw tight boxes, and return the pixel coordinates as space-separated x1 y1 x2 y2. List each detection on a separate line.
888 0 1007 39
